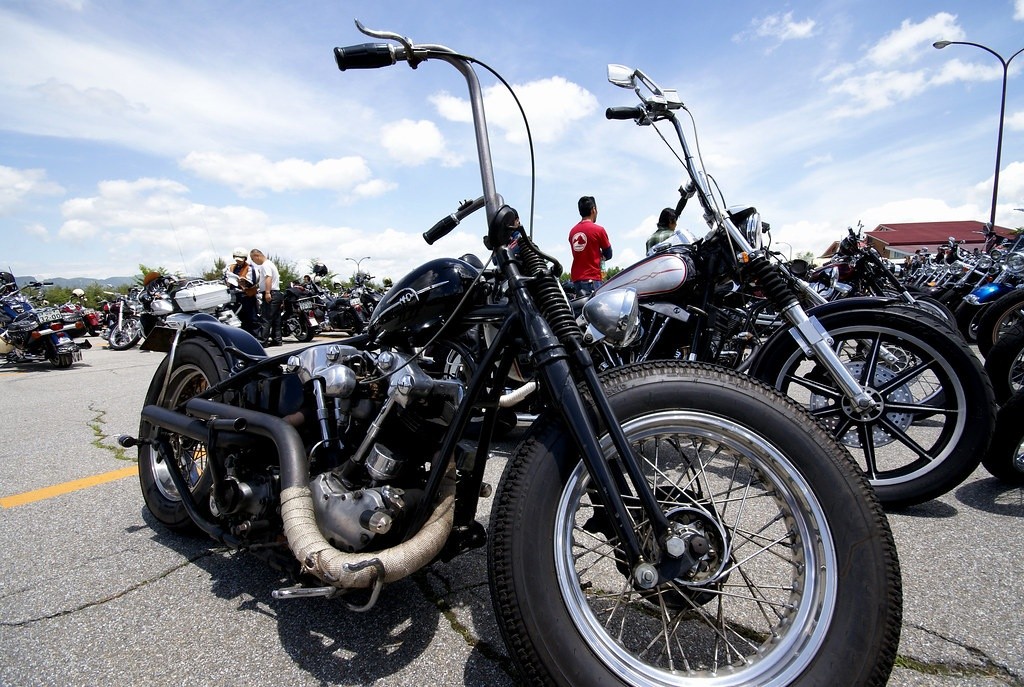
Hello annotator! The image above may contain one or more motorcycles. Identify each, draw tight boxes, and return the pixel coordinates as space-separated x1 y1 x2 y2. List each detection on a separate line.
0 265 394 373
522 228 1024 492
117 16 905 687
422 61 999 517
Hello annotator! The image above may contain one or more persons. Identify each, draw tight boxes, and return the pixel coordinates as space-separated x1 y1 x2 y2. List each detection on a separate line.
646 207 677 252
220 247 259 338
569 196 612 295
508 209 523 255
292 275 312 289
249 249 283 348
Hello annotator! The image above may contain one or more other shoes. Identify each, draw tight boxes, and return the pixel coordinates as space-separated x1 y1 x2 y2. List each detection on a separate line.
268 340 282 347
260 341 270 347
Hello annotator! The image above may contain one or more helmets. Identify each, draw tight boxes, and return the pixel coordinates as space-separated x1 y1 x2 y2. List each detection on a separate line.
232 245 248 262
313 263 328 277
72 288 85 298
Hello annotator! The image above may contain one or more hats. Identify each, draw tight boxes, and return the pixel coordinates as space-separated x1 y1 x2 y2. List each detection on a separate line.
234 257 246 262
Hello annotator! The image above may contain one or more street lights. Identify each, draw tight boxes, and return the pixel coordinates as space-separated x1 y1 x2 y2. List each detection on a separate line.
933 39 1024 235
345 256 371 273
